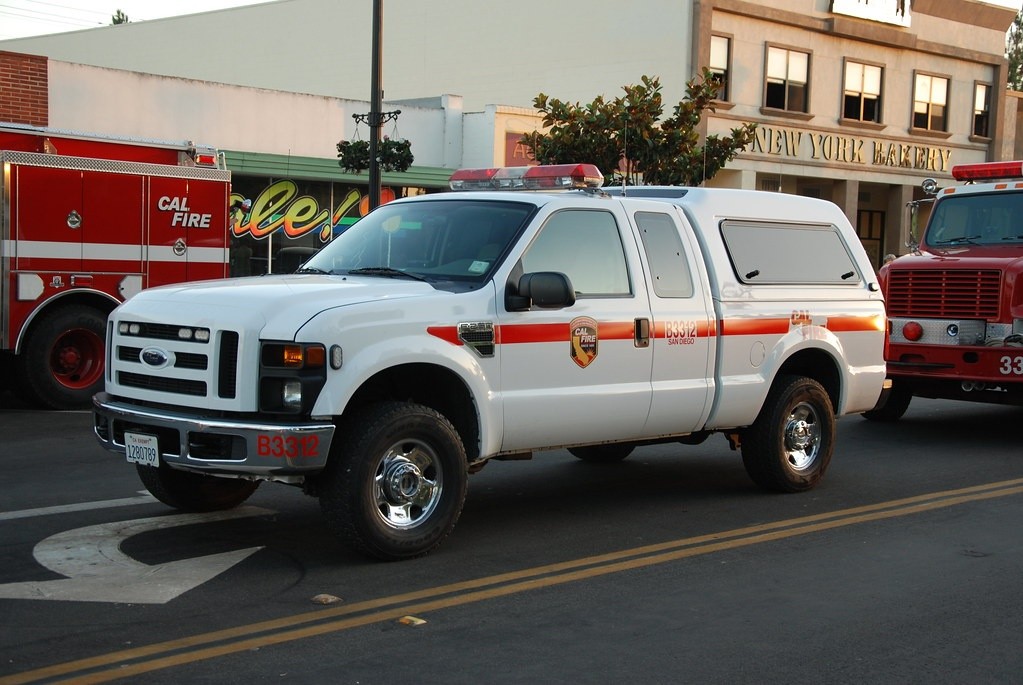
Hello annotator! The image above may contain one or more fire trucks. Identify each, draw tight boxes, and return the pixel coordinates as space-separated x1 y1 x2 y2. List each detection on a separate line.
0 123 232 405
861 158 1022 426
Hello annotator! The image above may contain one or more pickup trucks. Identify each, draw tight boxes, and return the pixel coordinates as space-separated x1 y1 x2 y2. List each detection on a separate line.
92 164 893 559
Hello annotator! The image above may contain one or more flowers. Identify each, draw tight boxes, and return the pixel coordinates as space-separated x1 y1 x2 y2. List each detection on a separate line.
336 140 370 175
375 135 414 173
